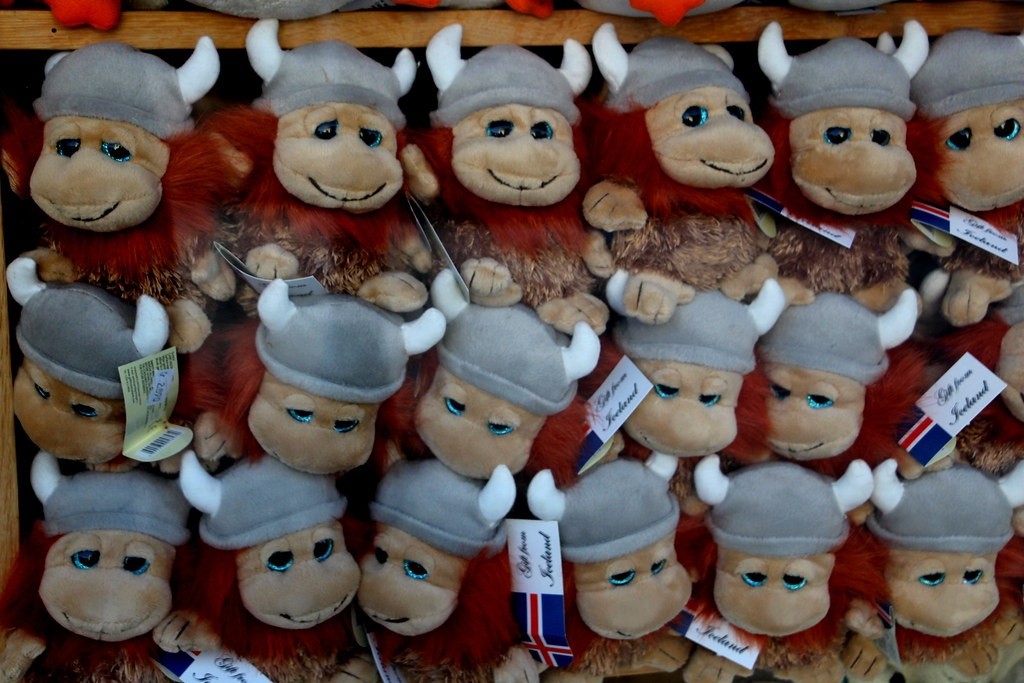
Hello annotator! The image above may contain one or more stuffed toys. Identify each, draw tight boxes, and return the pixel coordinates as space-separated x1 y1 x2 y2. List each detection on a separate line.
0 1 1023 683
